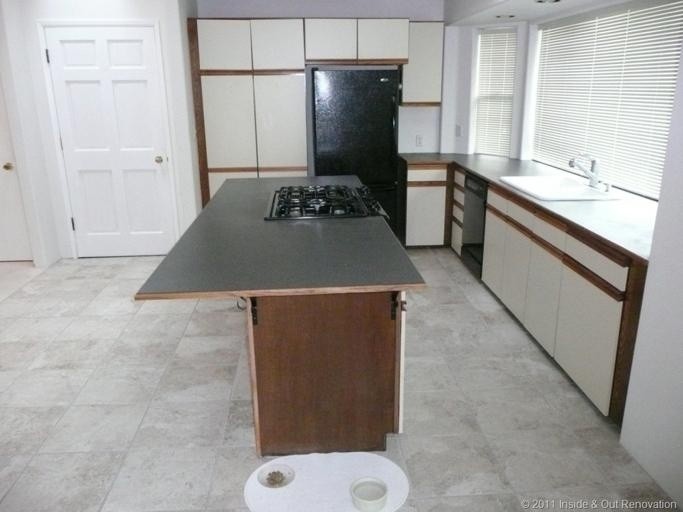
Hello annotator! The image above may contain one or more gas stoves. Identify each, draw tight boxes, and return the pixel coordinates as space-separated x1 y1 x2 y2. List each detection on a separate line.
265 183 390 221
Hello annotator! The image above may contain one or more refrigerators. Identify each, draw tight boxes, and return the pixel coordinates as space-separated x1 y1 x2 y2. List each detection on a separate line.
311 65 399 237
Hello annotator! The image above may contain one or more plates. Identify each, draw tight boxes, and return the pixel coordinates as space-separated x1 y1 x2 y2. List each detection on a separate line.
256 462 296 489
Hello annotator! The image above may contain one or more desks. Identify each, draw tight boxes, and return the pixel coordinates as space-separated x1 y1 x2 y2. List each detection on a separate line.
135 172 428 457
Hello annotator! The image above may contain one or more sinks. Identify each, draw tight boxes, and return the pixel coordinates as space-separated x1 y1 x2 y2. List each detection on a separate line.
500 172 619 202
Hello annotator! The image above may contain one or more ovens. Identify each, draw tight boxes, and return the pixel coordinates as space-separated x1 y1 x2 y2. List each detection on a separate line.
459 172 489 280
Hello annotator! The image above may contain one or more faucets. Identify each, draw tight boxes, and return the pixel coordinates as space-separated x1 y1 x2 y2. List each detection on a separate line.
569 149 602 188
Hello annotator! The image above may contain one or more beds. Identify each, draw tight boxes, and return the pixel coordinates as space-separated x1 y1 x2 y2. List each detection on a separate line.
244 449 411 512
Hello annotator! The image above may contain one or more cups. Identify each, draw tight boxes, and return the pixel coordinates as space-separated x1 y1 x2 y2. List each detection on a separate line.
349 476 388 512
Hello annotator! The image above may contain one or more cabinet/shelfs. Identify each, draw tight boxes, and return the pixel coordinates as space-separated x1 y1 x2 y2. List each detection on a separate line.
188 18 306 73
192 74 308 207
551 226 630 417
482 185 507 299
449 166 466 258
407 162 447 249
502 196 535 325
305 18 410 64
396 20 443 107
523 211 568 359
